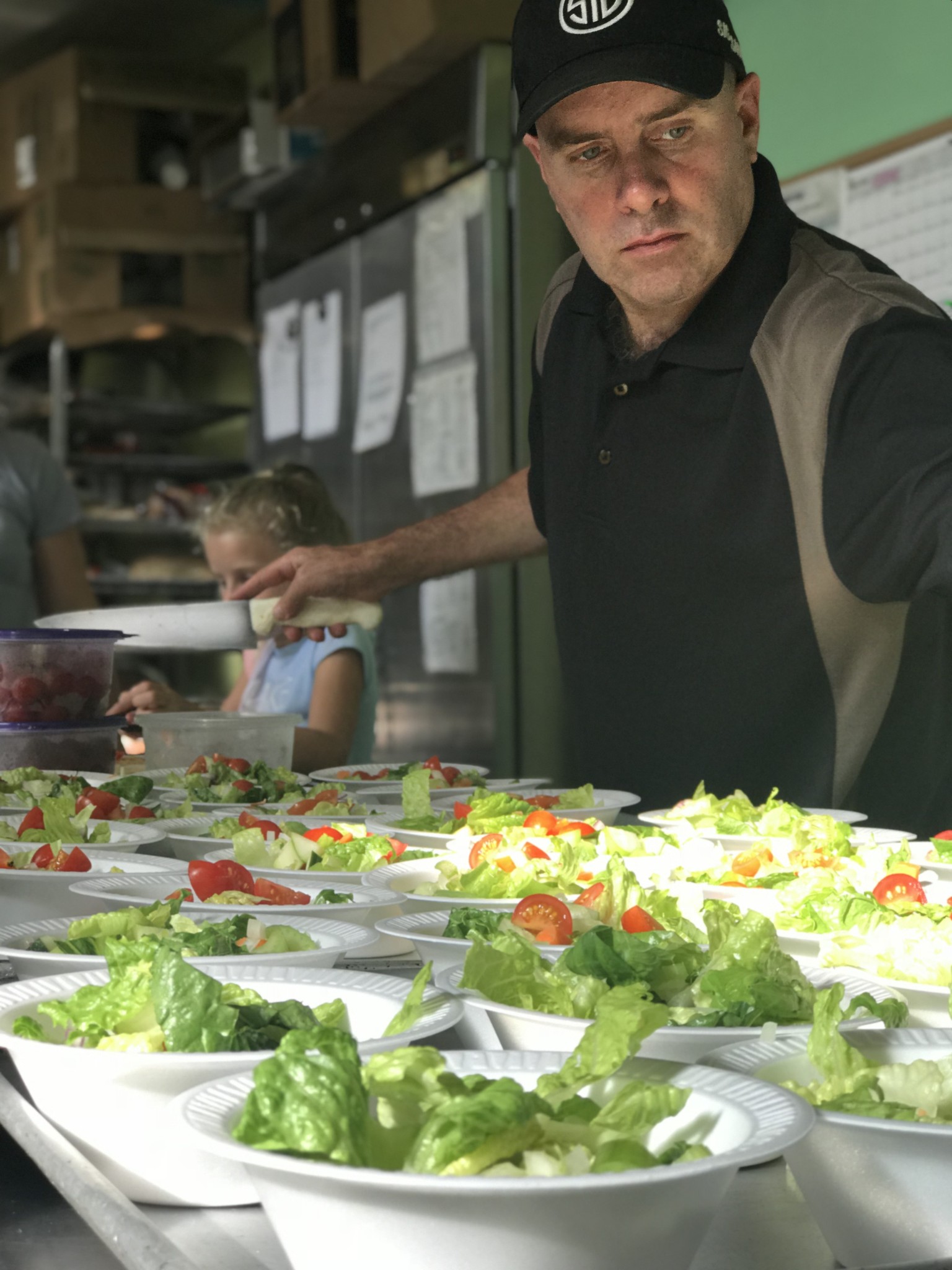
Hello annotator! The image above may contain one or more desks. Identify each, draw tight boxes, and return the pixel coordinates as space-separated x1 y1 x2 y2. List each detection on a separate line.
0 951 834 1270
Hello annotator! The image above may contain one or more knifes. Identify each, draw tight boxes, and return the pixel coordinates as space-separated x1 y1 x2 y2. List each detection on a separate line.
33 595 383 649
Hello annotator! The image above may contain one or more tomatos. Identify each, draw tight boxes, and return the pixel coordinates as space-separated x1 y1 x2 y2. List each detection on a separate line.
0 755 826 945
869 873 928 906
934 830 952 840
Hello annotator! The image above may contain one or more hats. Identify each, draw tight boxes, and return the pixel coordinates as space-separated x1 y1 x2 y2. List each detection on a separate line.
510 0 747 141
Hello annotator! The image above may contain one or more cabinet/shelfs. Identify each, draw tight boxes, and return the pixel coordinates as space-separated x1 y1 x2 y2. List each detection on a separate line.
42 327 269 604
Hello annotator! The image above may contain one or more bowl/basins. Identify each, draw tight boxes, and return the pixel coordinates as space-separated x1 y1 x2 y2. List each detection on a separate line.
0 762 951 1270
134 709 303 771
0 715 131 775
0 628 139 723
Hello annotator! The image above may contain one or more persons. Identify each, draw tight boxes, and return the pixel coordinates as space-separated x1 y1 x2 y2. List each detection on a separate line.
228 0 952 845
104 461 378 777
0 402 99 640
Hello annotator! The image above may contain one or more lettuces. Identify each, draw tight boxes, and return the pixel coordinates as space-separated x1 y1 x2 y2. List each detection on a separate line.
233 1019 951 1178
11 889 357 1048
0 754 952 872
382 860 951 1038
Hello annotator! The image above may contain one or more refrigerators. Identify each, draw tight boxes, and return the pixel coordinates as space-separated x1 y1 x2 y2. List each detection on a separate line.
227 36 576 787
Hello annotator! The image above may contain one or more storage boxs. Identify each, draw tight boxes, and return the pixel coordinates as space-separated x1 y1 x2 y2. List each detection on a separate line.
0 39 255 225
355 0 484 91
0 625 139 721
263 0 412 139
131 709 299 788
0 724 123 775
0 183 260 354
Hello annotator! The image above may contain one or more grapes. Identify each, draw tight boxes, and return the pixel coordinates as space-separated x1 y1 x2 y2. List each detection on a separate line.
0 646 110 724
